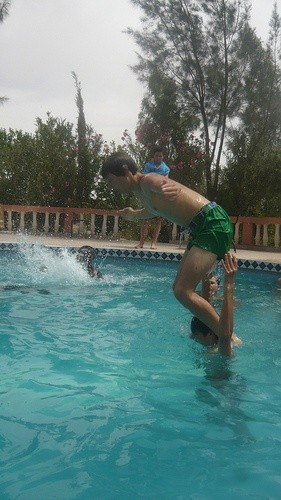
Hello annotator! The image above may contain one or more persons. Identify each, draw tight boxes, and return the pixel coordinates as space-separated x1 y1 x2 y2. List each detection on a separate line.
199 272 241 309
101 150 242 353
134 147 170 249
190 315 256 443
75 246 103 279
